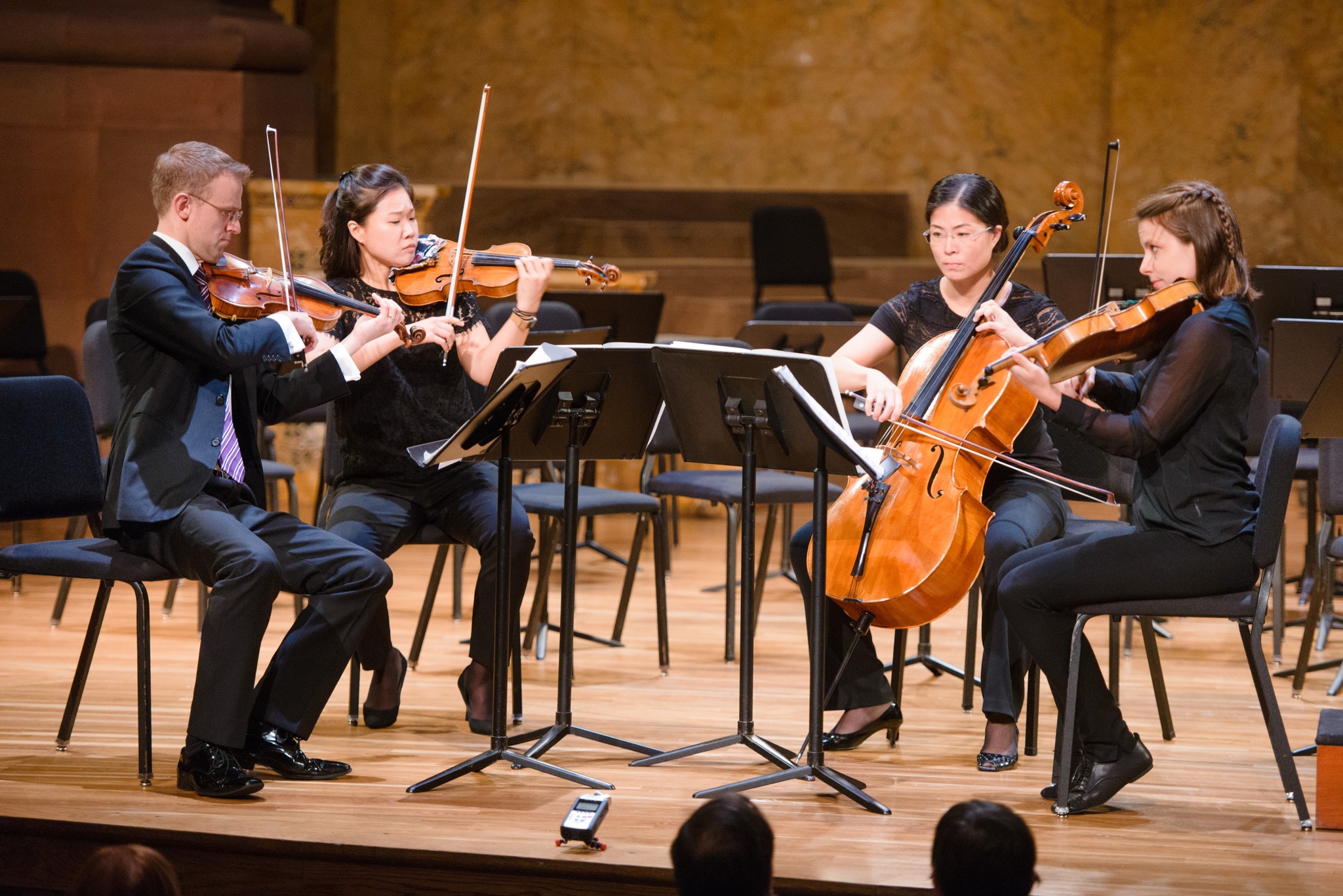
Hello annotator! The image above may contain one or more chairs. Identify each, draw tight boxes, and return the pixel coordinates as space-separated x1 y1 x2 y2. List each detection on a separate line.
0 206 1343 830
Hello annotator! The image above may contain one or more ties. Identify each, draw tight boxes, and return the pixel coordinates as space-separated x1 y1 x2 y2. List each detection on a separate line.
193 266 247 486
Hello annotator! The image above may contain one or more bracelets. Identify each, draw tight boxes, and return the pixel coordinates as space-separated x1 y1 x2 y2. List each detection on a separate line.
511 306 539 320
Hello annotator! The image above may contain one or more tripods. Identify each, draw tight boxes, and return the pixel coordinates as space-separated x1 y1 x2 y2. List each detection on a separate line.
1244 265 1343 696
404 289 892 816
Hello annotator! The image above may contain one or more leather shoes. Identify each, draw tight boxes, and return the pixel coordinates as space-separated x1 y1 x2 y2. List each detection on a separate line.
177 742 266 795
1041 747 1084 800
1056 732 1155 814
237 723 356 779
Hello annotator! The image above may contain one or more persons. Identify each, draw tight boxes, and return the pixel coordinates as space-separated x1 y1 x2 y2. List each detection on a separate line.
671 794 773 896
931 800 1041 896
317 166 553 736
72 845 180 896
101 142 405 796
998 182 1264 815
791 170 1074 771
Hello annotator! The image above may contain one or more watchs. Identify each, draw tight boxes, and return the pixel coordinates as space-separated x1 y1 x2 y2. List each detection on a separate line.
510 313 538 331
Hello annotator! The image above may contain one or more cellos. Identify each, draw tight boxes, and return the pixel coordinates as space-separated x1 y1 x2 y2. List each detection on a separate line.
789 179 1088 768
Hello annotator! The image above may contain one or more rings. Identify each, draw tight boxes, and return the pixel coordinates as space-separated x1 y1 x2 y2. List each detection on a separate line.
387 314 396 321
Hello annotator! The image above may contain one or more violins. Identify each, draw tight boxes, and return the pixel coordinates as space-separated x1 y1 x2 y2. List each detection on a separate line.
393 237 621 309
201 249 426 348
948 280 1205 413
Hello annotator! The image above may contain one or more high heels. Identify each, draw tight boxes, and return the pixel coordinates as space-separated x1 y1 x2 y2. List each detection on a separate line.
822 703 903 750
364 653 408 730
977 726 1020 770
455 667 495 735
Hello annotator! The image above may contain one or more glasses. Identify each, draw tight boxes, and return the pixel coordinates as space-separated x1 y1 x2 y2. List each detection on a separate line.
180 195 242 226
922 225 995 247
